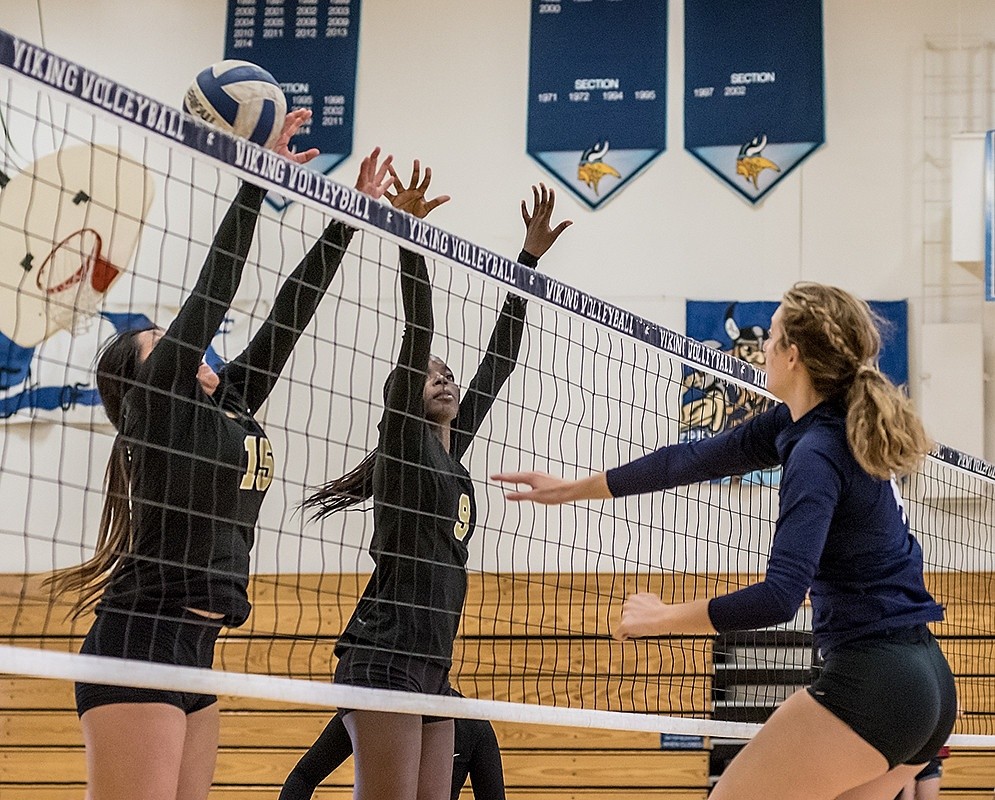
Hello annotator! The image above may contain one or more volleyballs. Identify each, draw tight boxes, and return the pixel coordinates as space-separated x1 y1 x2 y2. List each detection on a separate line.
183 60 288 149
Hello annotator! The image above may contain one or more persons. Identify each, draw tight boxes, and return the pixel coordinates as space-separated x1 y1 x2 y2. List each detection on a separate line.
273 158 578 799
491 281 958 800
34 109 399 800
